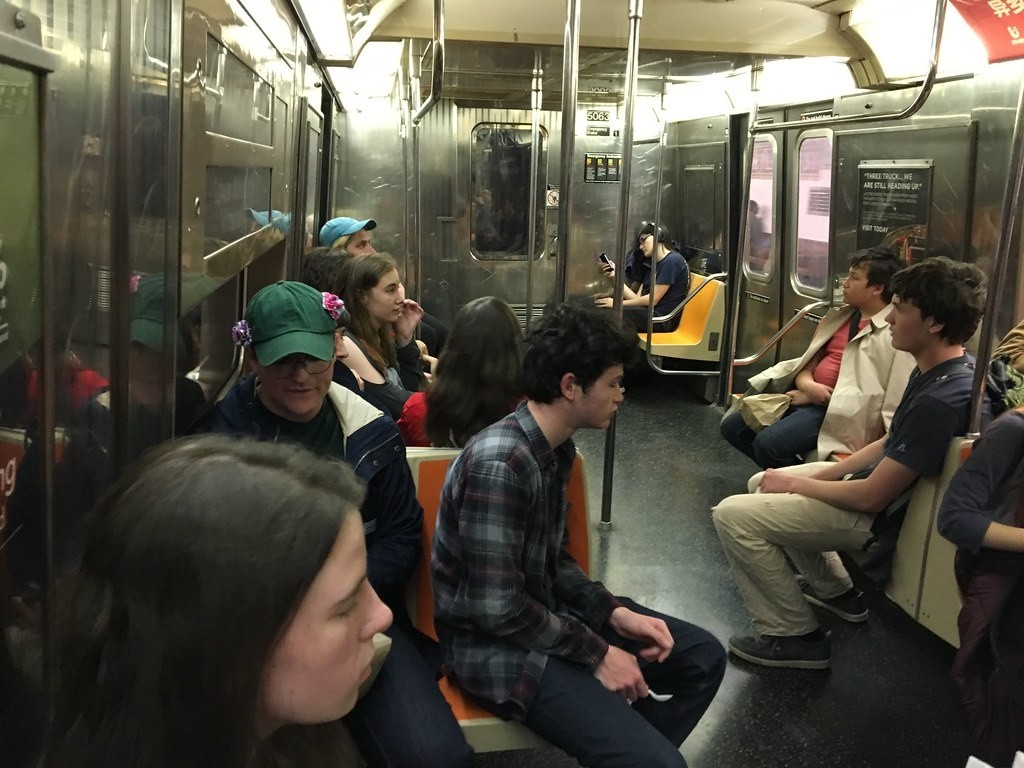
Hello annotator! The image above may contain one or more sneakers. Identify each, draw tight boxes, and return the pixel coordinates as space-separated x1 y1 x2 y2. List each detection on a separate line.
794 574 870 622
727 625 834 669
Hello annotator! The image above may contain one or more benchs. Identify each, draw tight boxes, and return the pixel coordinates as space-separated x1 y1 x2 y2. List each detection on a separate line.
635 272 726 363
375 441 595 753
730 390 980 654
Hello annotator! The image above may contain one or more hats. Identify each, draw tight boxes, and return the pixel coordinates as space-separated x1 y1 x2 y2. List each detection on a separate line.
244 280 337 367
126 274 182 353
318 216 376 246
243 207 281 227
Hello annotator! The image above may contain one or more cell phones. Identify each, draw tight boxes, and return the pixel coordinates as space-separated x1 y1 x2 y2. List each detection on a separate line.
600 253 614 273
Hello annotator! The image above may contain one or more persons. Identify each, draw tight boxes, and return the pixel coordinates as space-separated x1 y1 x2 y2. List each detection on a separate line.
748 200 762 270
711 258 992 666
594 222 691 394
476 148 529 255
34 432 395 768
937 409 1024 768
199 215 528 768
720 247 918 469
430 300 727 768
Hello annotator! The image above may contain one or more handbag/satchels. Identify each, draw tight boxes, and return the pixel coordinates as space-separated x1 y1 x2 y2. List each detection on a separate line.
985 359 1024 417
741 393 793 433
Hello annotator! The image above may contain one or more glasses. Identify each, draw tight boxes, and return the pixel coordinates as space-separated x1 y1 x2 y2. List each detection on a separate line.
638 233 651 244
265 355 332 379
